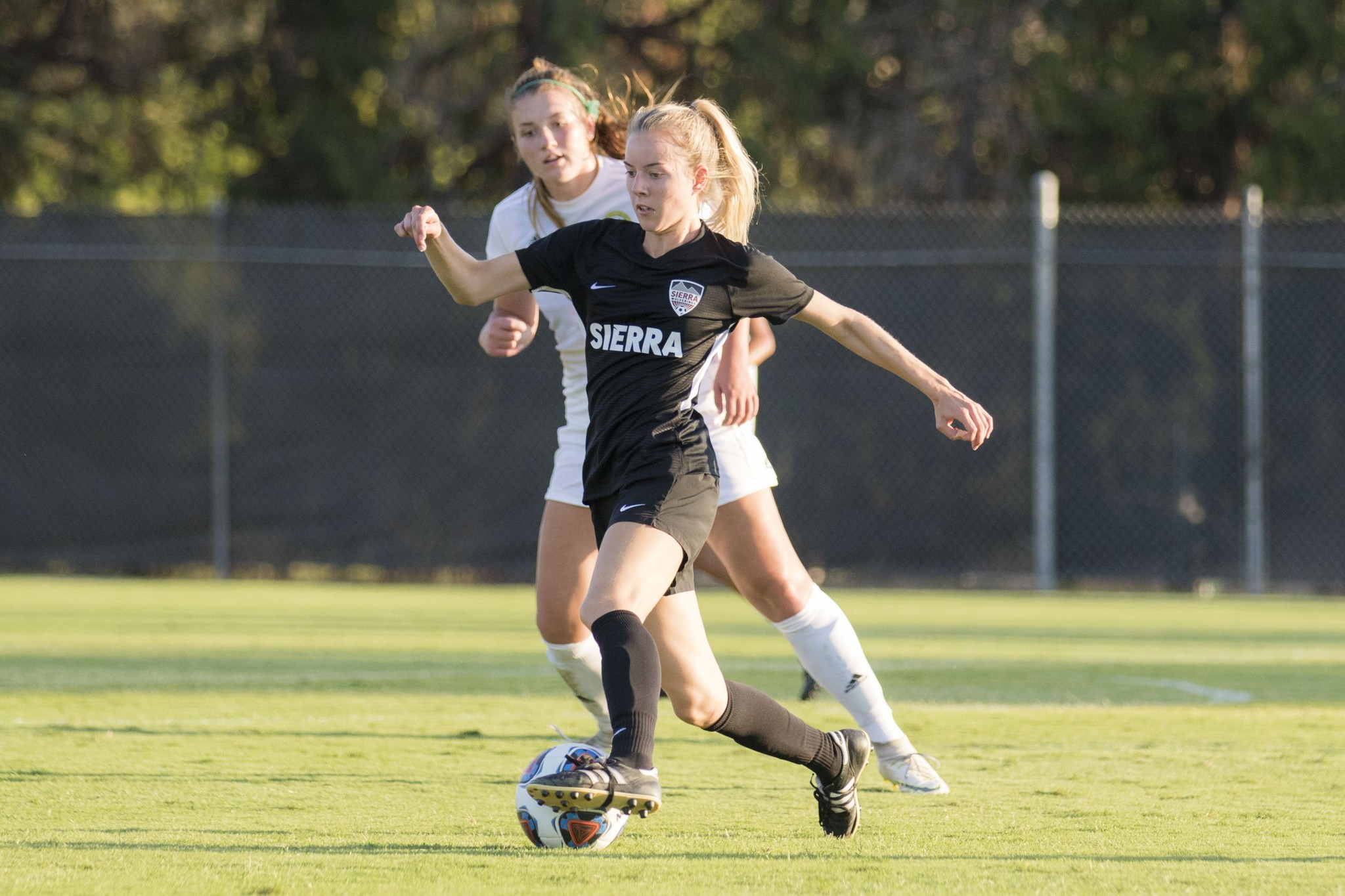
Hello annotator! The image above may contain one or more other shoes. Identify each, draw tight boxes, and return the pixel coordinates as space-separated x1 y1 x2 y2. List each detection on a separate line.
798 669 827 702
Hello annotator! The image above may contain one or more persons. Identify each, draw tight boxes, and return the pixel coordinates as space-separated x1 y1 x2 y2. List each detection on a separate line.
390 101 996 839
477 56 952 796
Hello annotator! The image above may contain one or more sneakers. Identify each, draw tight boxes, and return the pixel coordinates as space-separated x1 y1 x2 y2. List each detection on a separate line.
546 722 615 758
809 727 871 841
877 737 950 800
525 751 663 819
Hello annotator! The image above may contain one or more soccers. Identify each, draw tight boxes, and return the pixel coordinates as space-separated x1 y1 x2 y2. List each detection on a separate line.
515 743 626 849
